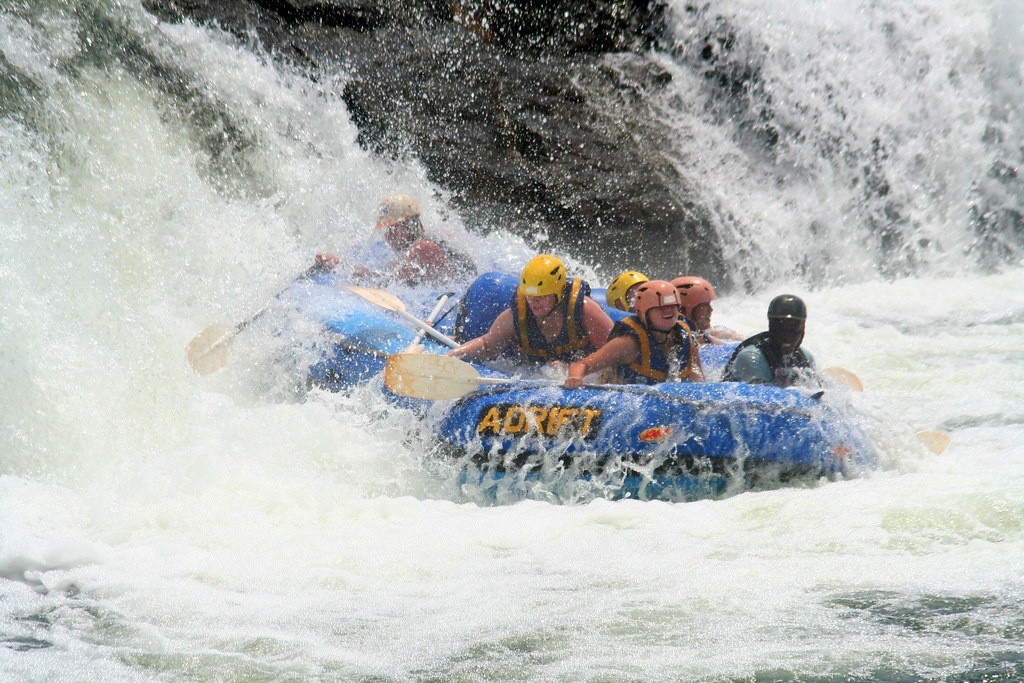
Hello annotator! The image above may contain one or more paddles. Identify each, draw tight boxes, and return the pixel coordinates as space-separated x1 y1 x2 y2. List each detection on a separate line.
336 282 486 391
807 358 862 400
184 263 319 376
379 349 658 403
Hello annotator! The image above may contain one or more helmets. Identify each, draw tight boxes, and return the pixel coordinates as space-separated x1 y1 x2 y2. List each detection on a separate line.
635 280 681 330
606 270 650 311
671 276 716 323
375 194 421 228
520 253 567 303
768 294 806 321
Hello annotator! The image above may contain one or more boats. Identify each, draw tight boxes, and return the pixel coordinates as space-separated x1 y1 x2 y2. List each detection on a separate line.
265 238 881 505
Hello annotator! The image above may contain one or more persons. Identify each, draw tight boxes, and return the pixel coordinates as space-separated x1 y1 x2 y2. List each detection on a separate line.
315 194 478 289
443 254 823 390
